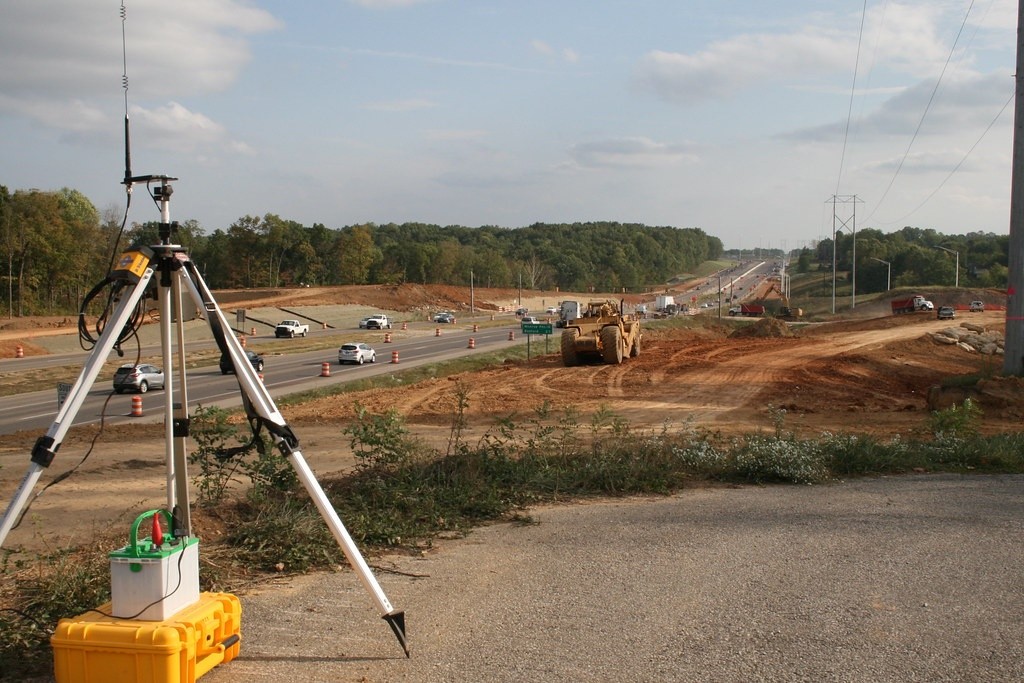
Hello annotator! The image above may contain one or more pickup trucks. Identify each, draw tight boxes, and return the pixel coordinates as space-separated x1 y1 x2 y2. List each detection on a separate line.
546 307 556 313
968 300 984 312
937 306 955 320
275 319 309 338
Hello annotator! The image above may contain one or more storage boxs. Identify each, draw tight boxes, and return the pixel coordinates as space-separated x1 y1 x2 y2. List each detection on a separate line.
50 591 242 683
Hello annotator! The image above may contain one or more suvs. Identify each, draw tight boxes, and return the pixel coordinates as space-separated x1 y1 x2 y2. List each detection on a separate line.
112 362 164 393
219 351 264 374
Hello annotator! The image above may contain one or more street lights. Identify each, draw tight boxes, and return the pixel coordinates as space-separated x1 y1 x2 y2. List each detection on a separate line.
870 257 891 291
932 245 959 288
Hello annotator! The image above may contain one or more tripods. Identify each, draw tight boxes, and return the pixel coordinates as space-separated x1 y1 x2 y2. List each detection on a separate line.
0 175 409 658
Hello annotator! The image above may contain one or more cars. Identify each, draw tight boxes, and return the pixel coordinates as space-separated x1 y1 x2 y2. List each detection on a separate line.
433 313 454 324
338 343 376 365
516 308 528 315
359 317 371 328
664 275 760 316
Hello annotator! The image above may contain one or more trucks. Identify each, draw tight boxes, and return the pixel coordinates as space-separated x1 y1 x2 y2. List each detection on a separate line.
655 296 675 311
635 306 647 315
890 295 934 313
774 306 803 321
727 302 765 316
367 315 394 330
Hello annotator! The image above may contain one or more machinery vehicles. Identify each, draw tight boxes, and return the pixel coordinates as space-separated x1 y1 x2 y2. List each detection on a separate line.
561 299 640 366
